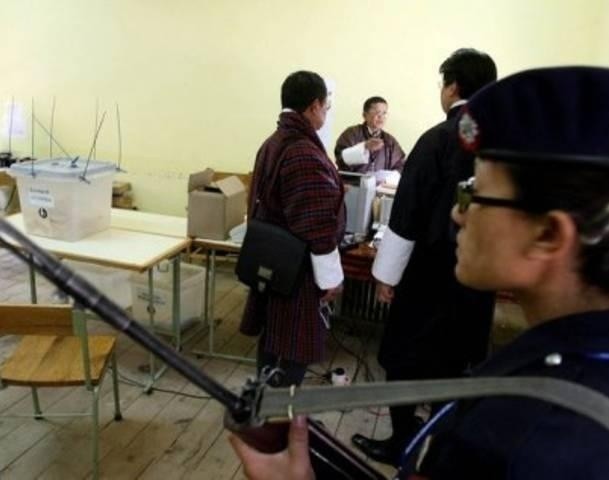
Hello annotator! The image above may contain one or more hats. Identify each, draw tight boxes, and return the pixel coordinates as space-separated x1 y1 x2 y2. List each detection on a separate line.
450 66 609 166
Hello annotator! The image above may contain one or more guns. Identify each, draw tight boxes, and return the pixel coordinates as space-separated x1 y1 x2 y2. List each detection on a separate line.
1 221 393 479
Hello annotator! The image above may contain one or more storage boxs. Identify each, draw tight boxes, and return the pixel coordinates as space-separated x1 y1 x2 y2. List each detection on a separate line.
186 167 246 243
9 156 115 240
339 169 376 237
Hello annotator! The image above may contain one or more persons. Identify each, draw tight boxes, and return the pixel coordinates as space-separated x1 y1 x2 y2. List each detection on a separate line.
237 70 348 389
334 96 405 187
353 48 499 462
227 61 609 480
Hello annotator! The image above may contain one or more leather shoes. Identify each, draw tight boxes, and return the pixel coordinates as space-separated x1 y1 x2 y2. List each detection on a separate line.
352 433 401 469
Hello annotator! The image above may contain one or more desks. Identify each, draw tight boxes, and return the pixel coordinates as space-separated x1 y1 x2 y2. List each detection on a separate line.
1 208 191 394
191 211 388 372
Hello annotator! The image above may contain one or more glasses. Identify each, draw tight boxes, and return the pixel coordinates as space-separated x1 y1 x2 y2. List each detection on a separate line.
458 175 541 214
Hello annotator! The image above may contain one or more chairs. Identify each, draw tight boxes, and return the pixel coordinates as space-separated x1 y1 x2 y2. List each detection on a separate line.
0 301 123 480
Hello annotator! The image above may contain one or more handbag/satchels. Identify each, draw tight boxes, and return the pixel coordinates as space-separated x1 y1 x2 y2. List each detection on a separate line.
233 219 308 297
